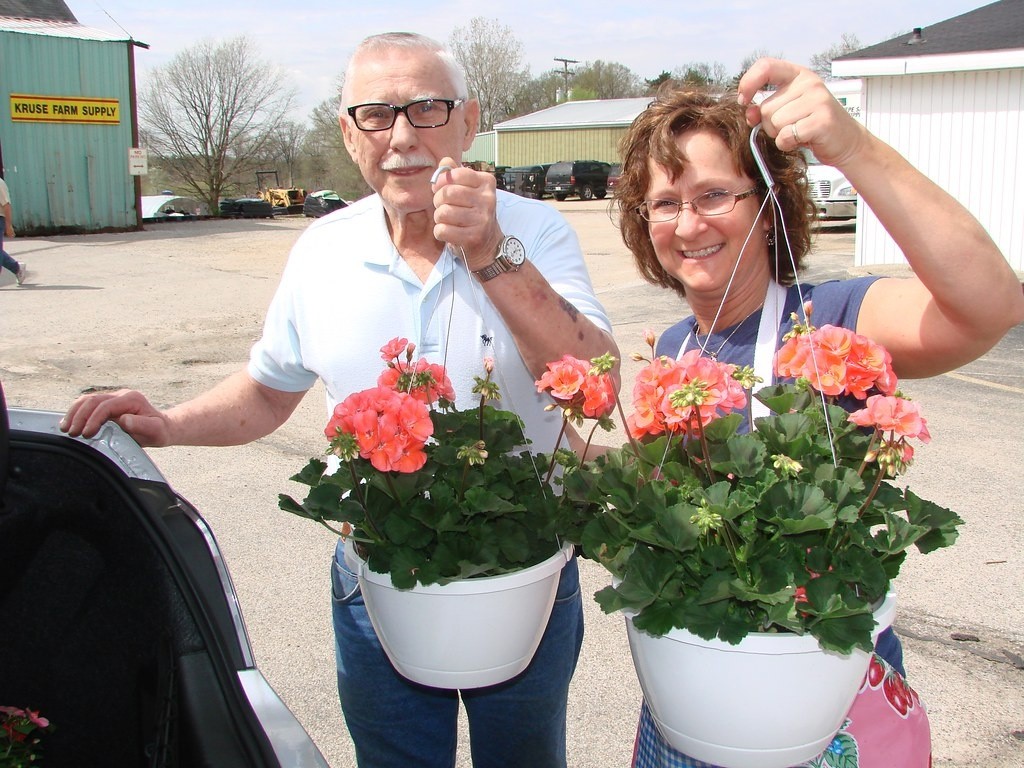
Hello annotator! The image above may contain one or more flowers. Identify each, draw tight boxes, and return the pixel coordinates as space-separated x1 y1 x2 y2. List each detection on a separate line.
275 339 610 574
1 706 56 768
592 305 966 652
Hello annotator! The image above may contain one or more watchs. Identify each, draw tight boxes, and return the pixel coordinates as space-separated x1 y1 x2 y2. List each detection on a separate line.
472 234 528 283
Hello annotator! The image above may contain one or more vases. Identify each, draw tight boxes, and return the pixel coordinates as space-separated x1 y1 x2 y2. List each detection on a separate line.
357 540 575 690
614 578 901 768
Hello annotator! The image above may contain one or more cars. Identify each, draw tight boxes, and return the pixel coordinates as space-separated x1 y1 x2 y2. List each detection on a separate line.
793 148 857 239
503 164 552 199
607 163 625 192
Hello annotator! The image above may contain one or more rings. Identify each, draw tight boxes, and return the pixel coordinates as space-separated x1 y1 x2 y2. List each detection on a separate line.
792 123 801 143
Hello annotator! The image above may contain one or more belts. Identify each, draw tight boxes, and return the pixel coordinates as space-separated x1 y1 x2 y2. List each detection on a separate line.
338 521 352 542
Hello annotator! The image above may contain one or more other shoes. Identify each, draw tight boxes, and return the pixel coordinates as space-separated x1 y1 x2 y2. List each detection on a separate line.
16 262 26 284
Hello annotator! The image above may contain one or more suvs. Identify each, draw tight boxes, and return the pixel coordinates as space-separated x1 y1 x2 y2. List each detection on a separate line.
544 159 610 201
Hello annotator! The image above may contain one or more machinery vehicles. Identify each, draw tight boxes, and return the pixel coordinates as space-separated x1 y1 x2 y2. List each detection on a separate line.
256 170 309 215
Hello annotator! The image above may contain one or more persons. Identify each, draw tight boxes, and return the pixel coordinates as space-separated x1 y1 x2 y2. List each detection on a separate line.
57 31 622 768
0 177 27 284
562 57 1024 768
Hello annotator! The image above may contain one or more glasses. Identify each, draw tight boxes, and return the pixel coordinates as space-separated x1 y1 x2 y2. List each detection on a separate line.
340 98 462 131
635 187 760 222
